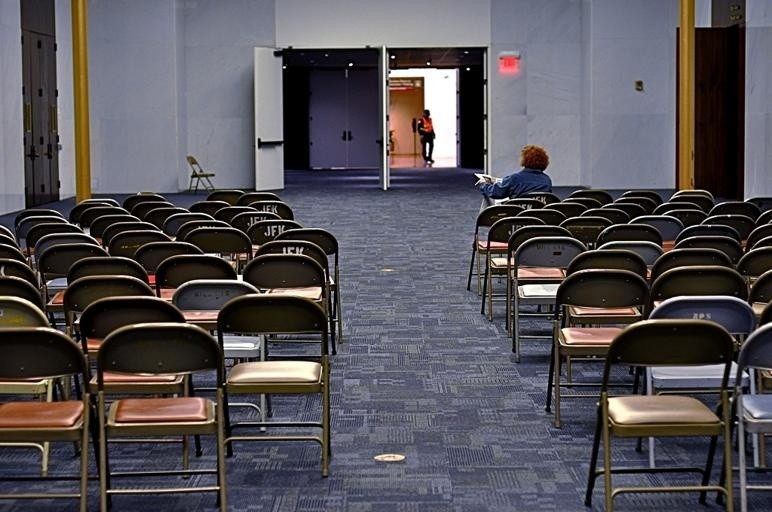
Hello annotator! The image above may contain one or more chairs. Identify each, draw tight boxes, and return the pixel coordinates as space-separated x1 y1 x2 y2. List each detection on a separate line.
97 321 229 510
1 326 91 510
186 156 215 193
583 320 736 511
216 294 332 476
701 326 771 511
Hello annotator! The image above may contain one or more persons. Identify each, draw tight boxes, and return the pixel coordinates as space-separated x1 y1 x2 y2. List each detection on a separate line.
417 110 435 163
479 144 552 200
